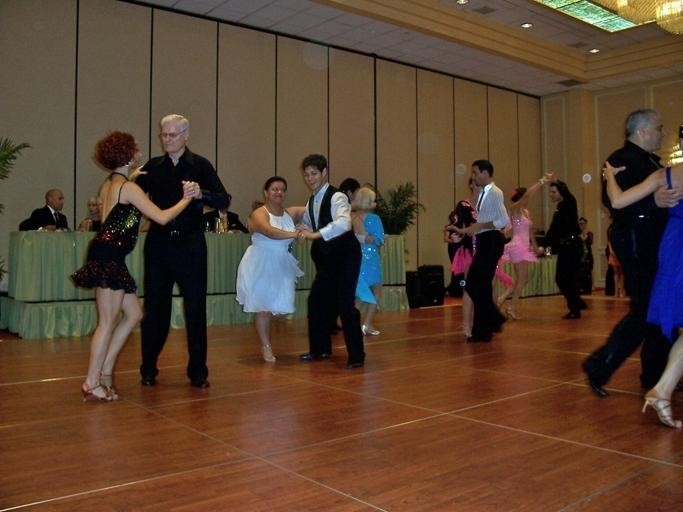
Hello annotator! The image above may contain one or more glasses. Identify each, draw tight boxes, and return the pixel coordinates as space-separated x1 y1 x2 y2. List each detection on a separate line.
159 129 185 137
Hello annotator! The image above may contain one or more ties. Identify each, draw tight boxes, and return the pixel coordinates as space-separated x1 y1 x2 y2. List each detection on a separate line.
305 193 319 234
477 191 485 212
51 212 61 225
650 157 661 169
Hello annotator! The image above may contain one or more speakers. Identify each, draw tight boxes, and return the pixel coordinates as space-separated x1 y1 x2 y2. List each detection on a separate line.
417 262 445 305
406 269 422 307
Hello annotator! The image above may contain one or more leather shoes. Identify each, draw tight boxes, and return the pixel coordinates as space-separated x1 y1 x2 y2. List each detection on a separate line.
346 360 364 369
562 310 580 319
467 334 491 343
141 376 156 387
191 378 210 389
581 361 608 397
300 352 328 361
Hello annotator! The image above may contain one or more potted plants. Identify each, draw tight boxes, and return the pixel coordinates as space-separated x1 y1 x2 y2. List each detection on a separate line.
363 178 427 236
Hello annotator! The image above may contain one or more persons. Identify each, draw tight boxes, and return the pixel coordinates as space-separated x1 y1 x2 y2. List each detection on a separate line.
443 201 516 342
203 193 250 234
506 170 555 320
134 115 232 388
444 211 464 298
338 178 360 207
581 109 682 396
297 154 366 369
236 176 306 362
350 188 385 336
68 131 193 403
78 195 100 231
534 171 587 320
19 189 68 231
579 217 593 295
608 243 626 298
465 159 512 342
600 124 683 429
605 244 615 296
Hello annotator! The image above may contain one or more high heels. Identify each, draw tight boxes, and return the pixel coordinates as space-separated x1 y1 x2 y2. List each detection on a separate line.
361 323 380 336
80 382 113 404
641 389 682 429
506 307 522 321
100 373 118 401
260 344 276 362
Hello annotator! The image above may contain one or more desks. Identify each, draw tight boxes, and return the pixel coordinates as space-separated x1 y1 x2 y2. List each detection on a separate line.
6 231 406 340
495 252 561 298
